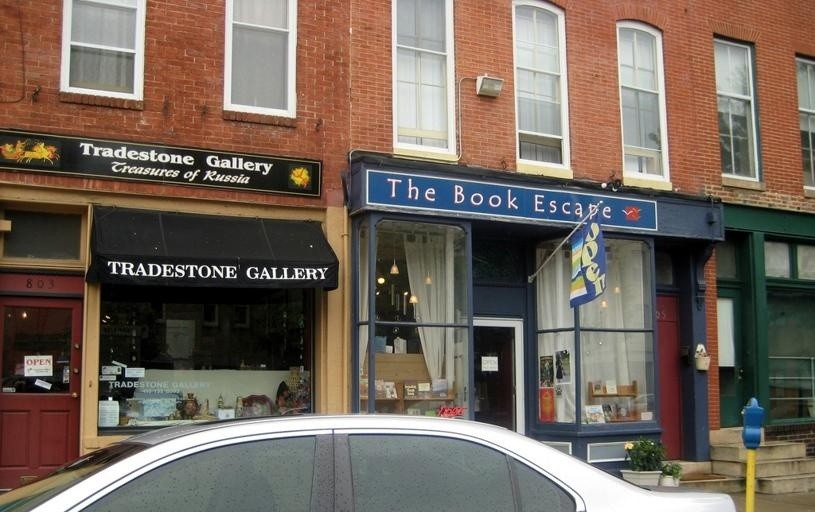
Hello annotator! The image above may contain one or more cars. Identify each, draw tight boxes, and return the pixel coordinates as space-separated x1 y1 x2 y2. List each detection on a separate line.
0 412 738 512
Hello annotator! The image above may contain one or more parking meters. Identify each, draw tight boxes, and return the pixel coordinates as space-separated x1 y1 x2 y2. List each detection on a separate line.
740 397 766 512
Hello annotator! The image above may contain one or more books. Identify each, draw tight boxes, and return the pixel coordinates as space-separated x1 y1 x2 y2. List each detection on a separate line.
359 378 449 416
605 380 617 394
590 380 603 395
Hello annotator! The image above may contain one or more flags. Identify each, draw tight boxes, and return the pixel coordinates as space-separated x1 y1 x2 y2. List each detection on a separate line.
568 211 608 308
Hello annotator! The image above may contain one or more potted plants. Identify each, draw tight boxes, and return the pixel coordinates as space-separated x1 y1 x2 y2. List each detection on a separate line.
619 434 681 488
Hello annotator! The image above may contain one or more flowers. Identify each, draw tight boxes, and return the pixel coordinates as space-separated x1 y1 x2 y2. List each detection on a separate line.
436 403 467 417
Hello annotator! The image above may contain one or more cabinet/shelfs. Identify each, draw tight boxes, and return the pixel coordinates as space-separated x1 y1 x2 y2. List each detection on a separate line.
588 381 639 422
360 353 455 417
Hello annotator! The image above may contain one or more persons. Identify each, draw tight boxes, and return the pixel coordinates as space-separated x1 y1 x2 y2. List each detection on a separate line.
544 360 552 383
556 354 564 382
591 412 603 421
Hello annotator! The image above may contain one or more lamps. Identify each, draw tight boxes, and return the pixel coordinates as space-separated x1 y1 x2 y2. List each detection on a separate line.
423 231 434 285
599 246 621 308
389 234 400 275
476 75 505 98
601 178 621 192
407 230 419 304
